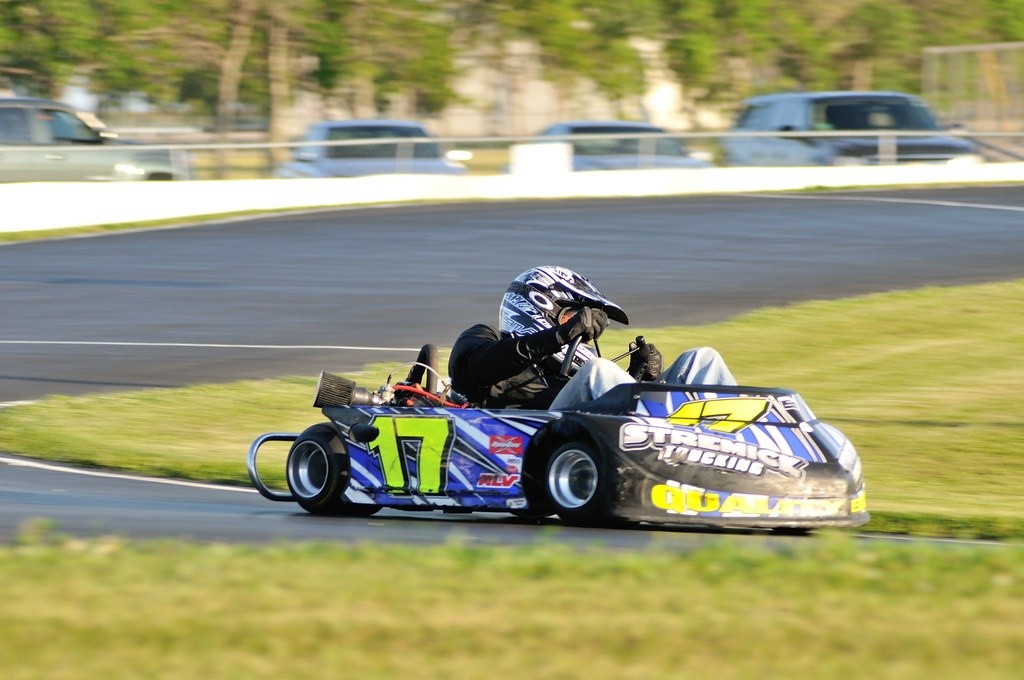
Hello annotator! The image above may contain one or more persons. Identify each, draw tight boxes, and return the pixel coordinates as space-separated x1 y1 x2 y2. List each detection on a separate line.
447 266 738 410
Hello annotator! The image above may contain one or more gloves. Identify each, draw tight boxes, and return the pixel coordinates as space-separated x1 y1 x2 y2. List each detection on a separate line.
558 306 609 342
626 341 662 382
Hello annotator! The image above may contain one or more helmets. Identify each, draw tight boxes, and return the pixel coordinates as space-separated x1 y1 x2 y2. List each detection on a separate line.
498 265 630 337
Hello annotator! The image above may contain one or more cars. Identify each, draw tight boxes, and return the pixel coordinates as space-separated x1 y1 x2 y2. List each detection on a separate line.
1 99 193 182
509 120 714 165
716 91 991 161
272 113 471 173
